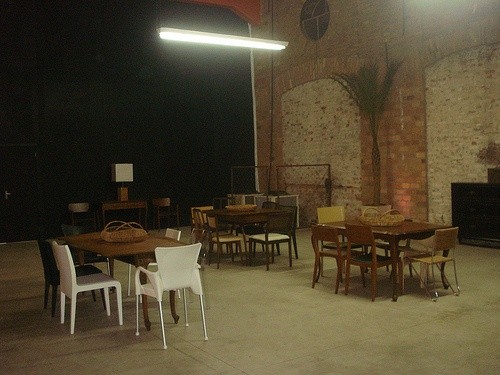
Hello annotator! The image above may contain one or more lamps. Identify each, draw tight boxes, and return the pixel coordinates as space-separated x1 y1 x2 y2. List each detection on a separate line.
156 0 289 50
112 163 133 202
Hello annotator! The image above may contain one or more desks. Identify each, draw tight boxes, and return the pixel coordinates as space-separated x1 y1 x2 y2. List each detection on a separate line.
98 201 148 230
315 219 452 302
202 208 287 259
57 232 186 332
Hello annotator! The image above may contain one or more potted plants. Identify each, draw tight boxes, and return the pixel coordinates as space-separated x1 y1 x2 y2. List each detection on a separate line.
477 141 500 182
326 59 403 215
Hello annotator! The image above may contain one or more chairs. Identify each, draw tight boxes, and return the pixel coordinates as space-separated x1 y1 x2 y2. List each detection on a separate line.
311 205 460 302
37 197 298 349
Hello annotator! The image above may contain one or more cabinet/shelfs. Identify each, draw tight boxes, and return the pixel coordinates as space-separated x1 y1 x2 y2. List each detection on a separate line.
451 182 500 248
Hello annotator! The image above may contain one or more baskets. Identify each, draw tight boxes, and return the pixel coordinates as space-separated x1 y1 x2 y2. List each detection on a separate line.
101 220 147 243
225 204 257 211
358 207 405 227
